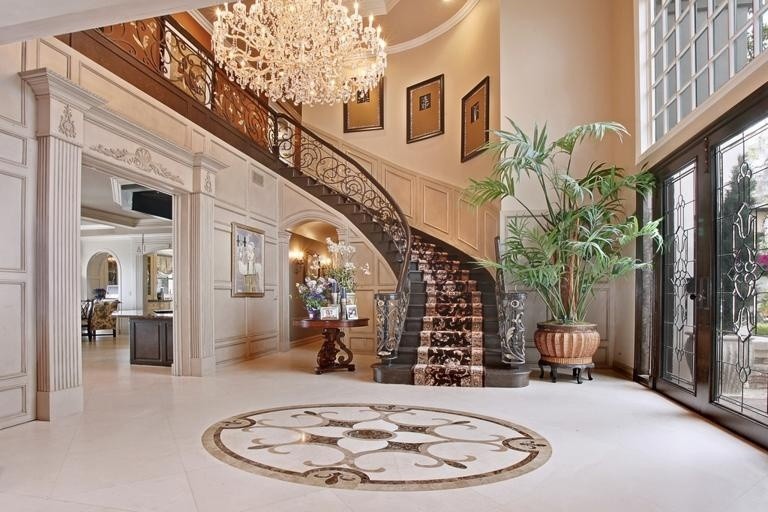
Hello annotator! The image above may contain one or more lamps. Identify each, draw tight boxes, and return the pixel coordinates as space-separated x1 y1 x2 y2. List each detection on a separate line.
211 0 387 106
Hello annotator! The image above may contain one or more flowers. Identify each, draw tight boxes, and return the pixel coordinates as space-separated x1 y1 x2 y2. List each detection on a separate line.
288 236 372 310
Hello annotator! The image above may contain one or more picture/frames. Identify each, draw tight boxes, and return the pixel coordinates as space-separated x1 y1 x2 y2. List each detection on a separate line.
343 73 384 132
406 74 444 144
459 76 492 163
230 221 266 298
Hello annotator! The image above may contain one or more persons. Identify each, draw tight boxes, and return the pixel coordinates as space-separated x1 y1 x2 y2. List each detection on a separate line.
348 307 352 318
350 308 357 318
330 310 335 317
325 310 331 317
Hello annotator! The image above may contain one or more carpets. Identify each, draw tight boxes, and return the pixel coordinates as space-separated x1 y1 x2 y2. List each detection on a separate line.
410 233 485 387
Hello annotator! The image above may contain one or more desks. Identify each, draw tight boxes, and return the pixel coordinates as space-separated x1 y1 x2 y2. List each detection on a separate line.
293 316 369 375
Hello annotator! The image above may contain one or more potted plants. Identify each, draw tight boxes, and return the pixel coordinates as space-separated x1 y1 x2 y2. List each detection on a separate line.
683 152 763 392
454 115 663 384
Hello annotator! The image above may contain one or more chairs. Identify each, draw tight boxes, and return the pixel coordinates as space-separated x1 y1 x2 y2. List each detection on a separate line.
81 299 120 341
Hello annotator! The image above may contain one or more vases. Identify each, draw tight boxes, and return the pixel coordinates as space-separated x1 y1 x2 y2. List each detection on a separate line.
306 293 356 319
